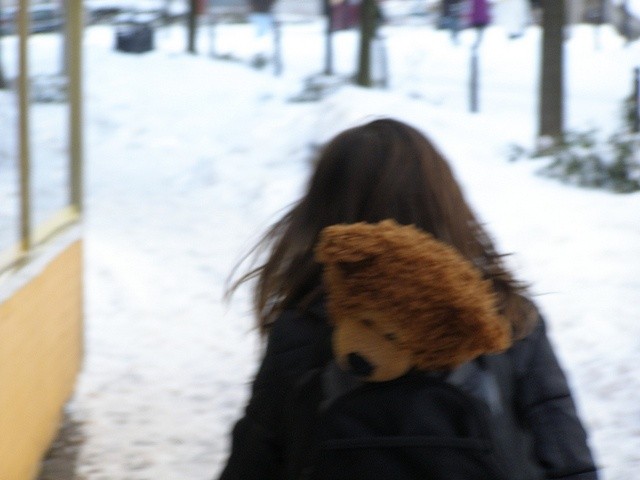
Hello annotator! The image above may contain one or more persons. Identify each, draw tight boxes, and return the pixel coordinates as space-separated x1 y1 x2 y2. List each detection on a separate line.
212 117 601 480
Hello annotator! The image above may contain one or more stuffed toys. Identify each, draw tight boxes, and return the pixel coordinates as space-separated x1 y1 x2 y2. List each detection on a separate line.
312 219 513 383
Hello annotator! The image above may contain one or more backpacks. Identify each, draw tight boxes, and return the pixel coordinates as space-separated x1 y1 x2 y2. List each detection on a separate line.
276 284 545 479
